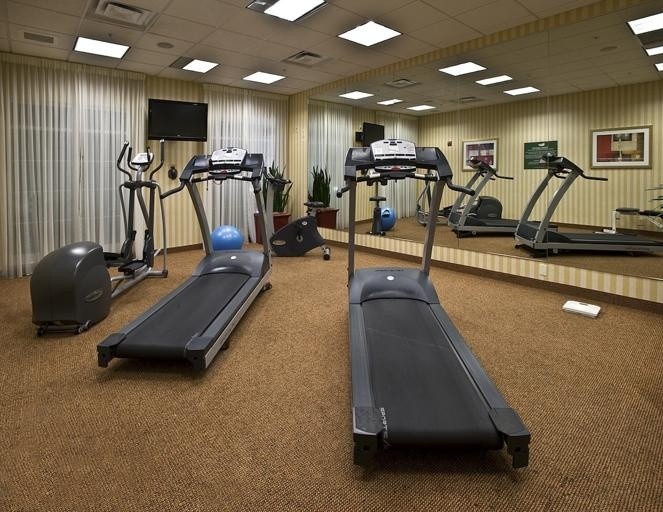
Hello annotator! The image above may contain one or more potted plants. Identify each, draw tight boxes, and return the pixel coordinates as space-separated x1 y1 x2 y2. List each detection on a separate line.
307 166 340 229
252 161 295 244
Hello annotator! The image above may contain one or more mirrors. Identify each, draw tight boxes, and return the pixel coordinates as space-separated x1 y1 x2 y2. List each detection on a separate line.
306 0 663 306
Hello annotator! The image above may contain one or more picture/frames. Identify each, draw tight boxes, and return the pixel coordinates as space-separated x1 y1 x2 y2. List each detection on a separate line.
460 137 500 171
586 125 653 170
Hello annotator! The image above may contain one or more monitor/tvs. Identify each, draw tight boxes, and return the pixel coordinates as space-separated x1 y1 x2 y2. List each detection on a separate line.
148 98 208 141
363 122 384 146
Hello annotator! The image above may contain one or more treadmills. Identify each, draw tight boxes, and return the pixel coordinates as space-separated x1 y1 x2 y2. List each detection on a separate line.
96 147 274 374
336 138 531 473
448 157 558 238
512 155 663 257
